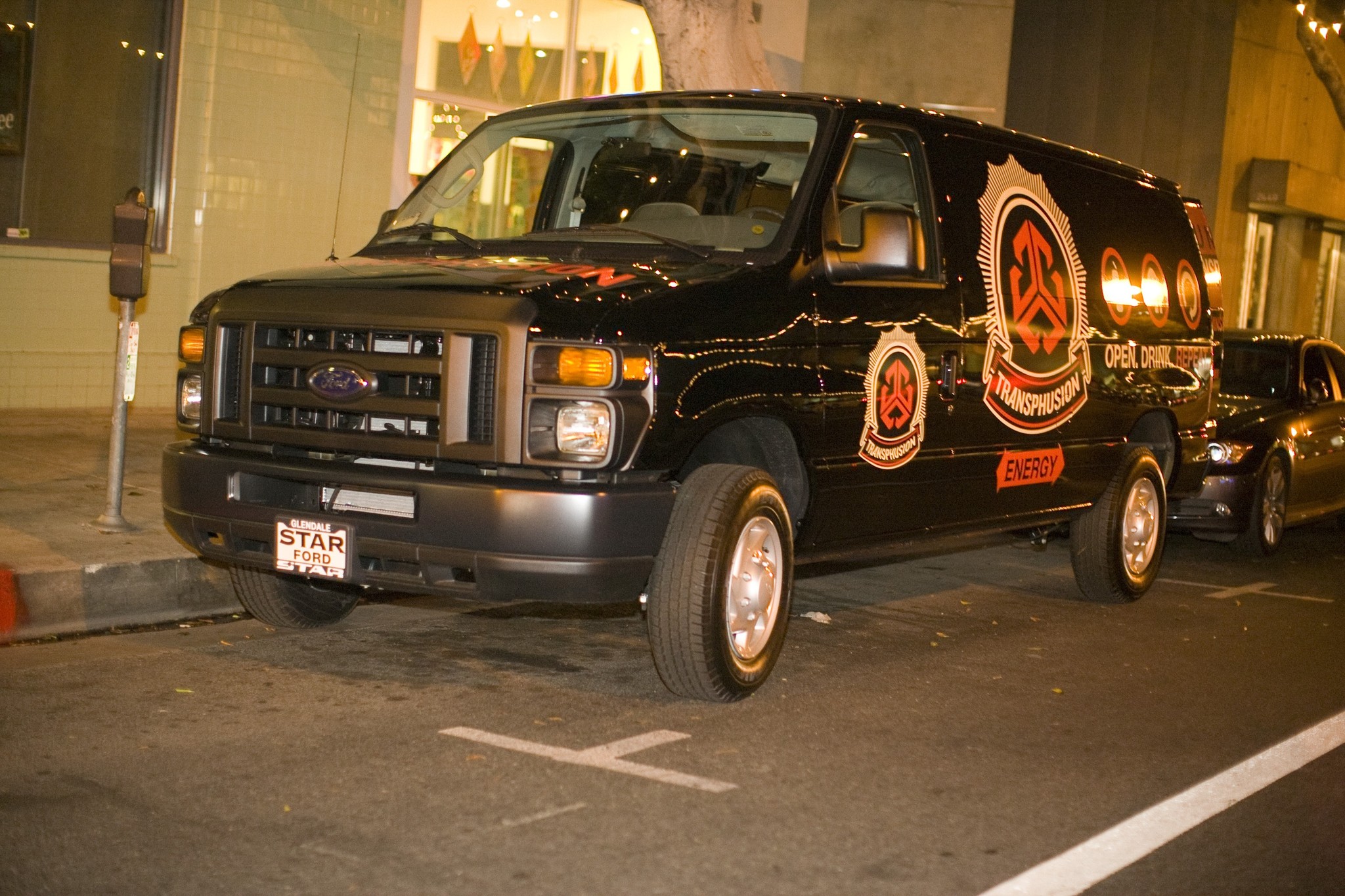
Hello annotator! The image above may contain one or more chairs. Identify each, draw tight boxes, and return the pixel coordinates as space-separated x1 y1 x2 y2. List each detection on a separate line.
840 200 909 246
630 203 698 222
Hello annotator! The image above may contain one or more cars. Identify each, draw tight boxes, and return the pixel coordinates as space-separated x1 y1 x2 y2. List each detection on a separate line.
1007 324 1345 561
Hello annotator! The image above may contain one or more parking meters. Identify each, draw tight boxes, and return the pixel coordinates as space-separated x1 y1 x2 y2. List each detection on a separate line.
89 186 157 532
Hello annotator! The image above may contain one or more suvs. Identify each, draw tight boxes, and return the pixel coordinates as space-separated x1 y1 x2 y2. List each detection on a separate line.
153 85 1223 702
